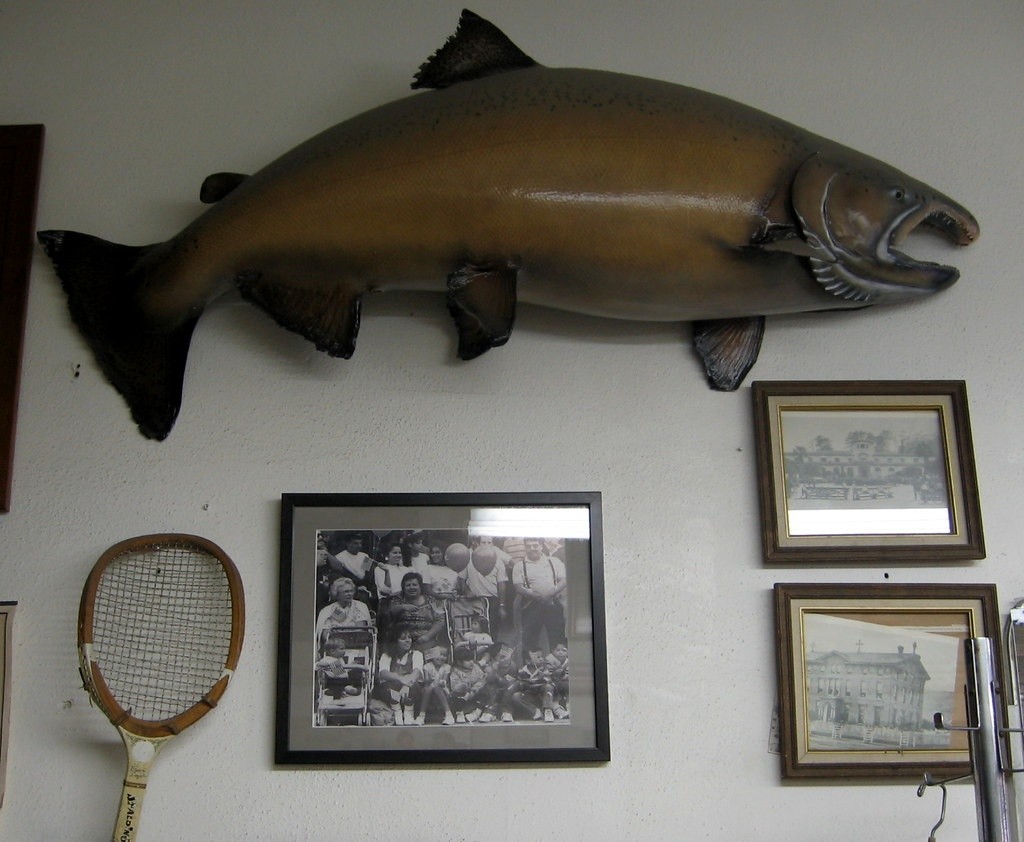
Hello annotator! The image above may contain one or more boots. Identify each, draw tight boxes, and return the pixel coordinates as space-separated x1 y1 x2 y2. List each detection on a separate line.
403 704 415 725
390 703 403 726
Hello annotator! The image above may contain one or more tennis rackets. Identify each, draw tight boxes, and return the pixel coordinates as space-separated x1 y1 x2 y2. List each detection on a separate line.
78 532 247 842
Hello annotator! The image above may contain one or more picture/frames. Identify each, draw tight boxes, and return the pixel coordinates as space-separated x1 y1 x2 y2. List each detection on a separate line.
768 580 1015 782
749 376 990 564
273 489 613 764
0 124 45 516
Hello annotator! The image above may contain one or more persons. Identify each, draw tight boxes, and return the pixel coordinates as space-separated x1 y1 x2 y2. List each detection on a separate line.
314 532 571 727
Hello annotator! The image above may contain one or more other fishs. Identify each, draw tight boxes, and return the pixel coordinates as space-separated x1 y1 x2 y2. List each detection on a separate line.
35 9 981 444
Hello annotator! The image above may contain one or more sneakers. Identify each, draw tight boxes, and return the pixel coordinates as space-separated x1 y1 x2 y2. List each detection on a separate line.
533 709 543 720
456 709 465 723
544 710 554 722
442 713 455 725
501 712 513 722
479 712 496 722
413 716 425 725
555 706 569 719
465 709 480 723
